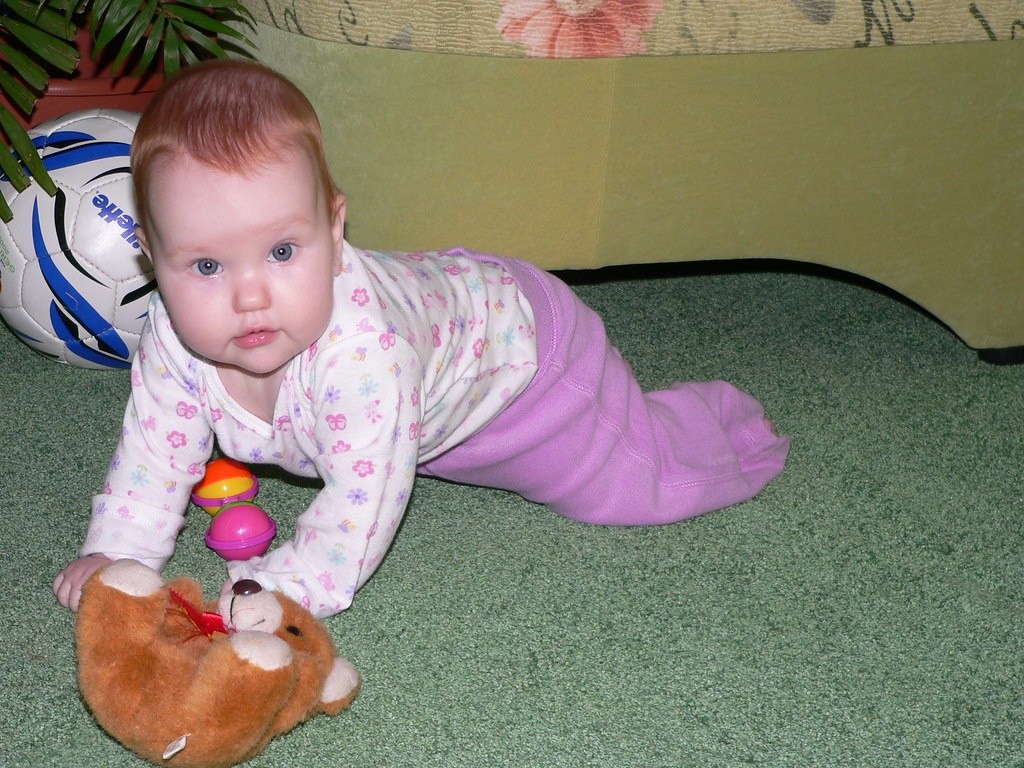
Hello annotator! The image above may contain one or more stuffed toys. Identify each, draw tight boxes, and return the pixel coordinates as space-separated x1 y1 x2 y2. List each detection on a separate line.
74 558 362 768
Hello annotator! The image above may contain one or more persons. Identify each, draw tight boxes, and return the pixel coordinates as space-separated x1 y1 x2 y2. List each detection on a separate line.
52 58 791 620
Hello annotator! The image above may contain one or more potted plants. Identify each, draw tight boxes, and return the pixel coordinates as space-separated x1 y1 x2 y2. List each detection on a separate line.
0 1 261 223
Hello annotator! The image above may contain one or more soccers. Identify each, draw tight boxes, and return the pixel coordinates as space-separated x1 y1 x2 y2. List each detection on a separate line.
0 107 162 371
